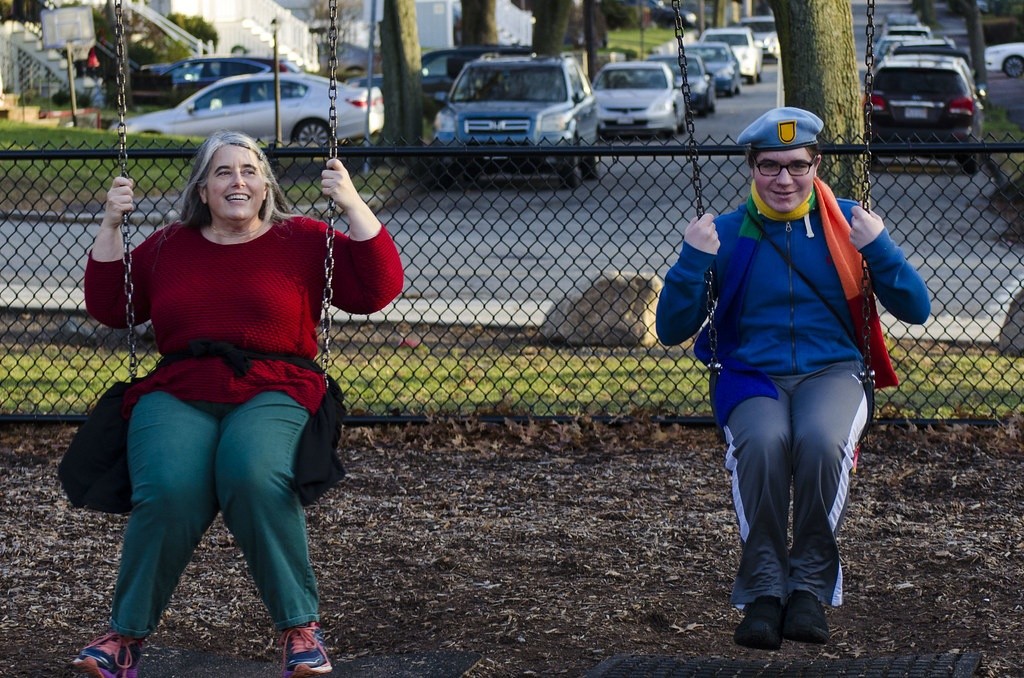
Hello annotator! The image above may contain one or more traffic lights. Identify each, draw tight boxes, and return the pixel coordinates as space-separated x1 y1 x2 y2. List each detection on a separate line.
84 45 105 73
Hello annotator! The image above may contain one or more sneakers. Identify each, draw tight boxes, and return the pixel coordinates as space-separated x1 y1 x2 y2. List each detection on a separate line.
69 629 143 678
781 590 830 645
733 595 784 651
278 622 333 678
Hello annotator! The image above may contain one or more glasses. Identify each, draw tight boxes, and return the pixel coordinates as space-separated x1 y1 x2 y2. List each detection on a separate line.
752 155 816 176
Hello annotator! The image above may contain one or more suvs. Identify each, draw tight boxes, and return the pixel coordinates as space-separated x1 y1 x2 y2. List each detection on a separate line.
430 51 599 191
341 43 534 123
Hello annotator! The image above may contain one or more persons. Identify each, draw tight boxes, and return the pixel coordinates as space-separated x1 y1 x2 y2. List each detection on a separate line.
656 107 931 650
70 132 403 678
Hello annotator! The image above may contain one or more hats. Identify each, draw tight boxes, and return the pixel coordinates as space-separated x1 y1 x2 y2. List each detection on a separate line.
736 107 824 149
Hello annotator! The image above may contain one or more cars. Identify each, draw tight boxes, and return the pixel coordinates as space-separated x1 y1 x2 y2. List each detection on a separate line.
725 17 781 68
640 52 717 118
107 71 385 149
102 55 305 108
316 39 382 76
697 27 767 86
606 0 714 31
683 42 742 98
860 13 988 174
591 60 685 140
983 41 1024 79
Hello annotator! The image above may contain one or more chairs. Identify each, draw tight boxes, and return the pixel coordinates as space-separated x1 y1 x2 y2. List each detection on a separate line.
610 75 627 88
649 75 665 87
